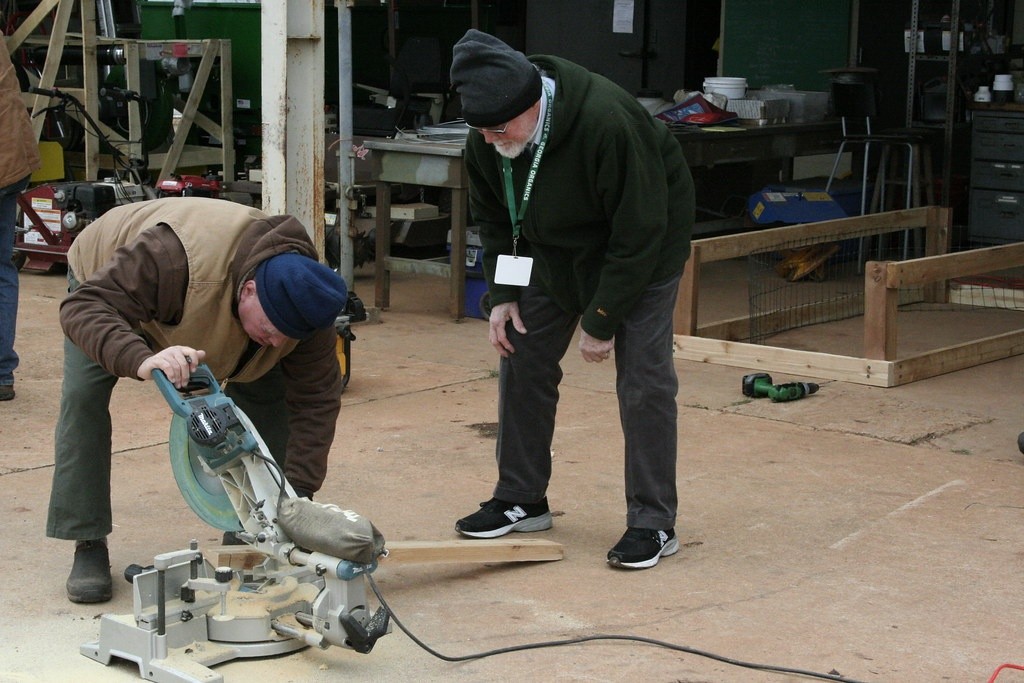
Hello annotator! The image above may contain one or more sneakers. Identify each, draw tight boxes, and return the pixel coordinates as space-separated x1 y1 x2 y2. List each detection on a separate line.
606 525 679 568
455 497 552 538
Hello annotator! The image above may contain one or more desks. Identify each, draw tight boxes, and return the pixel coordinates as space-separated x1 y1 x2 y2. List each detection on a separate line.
361 117 849 324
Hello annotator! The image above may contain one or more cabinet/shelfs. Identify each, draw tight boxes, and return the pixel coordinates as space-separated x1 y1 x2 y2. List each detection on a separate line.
967 107 1024 244
903 0 994 259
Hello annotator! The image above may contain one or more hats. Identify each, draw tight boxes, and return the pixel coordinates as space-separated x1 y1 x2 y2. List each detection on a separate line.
448 29 542 128
253 253 349 337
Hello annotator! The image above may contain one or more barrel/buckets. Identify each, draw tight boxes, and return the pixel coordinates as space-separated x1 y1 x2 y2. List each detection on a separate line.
703 77 749 99
636 89 665 115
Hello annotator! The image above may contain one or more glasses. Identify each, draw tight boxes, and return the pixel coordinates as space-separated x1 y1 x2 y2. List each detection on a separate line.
465 122 511 134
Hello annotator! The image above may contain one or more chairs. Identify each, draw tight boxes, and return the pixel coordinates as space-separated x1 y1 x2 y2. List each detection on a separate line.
823 74 923 275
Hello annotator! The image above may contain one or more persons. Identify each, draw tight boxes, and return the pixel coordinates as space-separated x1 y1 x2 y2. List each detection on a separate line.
45 197 348 602
449 29 696 569
0 29 42 400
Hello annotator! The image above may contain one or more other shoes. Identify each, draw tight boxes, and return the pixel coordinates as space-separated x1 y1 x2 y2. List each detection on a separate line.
219 530 248 545
0 384 15 401
65 539 113 603
